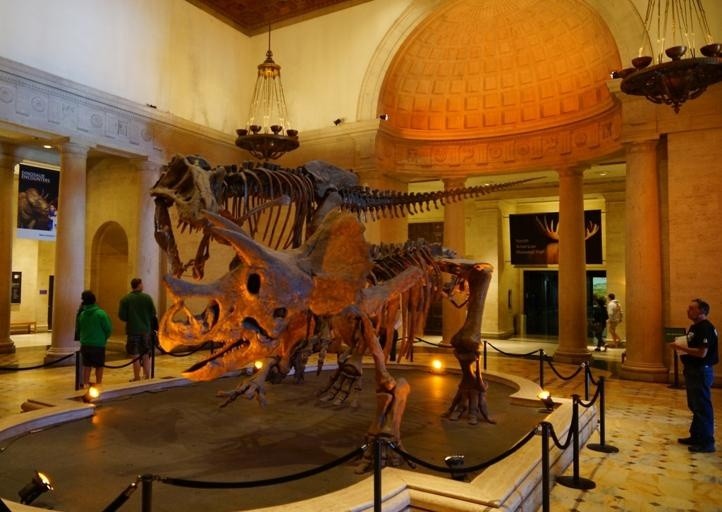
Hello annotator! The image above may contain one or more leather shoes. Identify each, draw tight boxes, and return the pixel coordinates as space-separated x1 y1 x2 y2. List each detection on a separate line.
678 436 703 444
604 344 608 352
595 348 601 351
688 444 715 453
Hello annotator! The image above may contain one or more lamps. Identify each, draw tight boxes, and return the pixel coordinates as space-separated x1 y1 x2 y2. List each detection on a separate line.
620 0 722 113
234 1 299 158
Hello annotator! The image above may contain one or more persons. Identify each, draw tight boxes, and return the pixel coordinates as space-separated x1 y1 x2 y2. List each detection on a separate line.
75 291 113 386
607 293 622 348
668 298 719 453
118 278 156 382
591 297 609 352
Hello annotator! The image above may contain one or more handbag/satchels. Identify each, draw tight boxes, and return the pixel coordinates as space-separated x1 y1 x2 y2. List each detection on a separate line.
591 320 603 331
